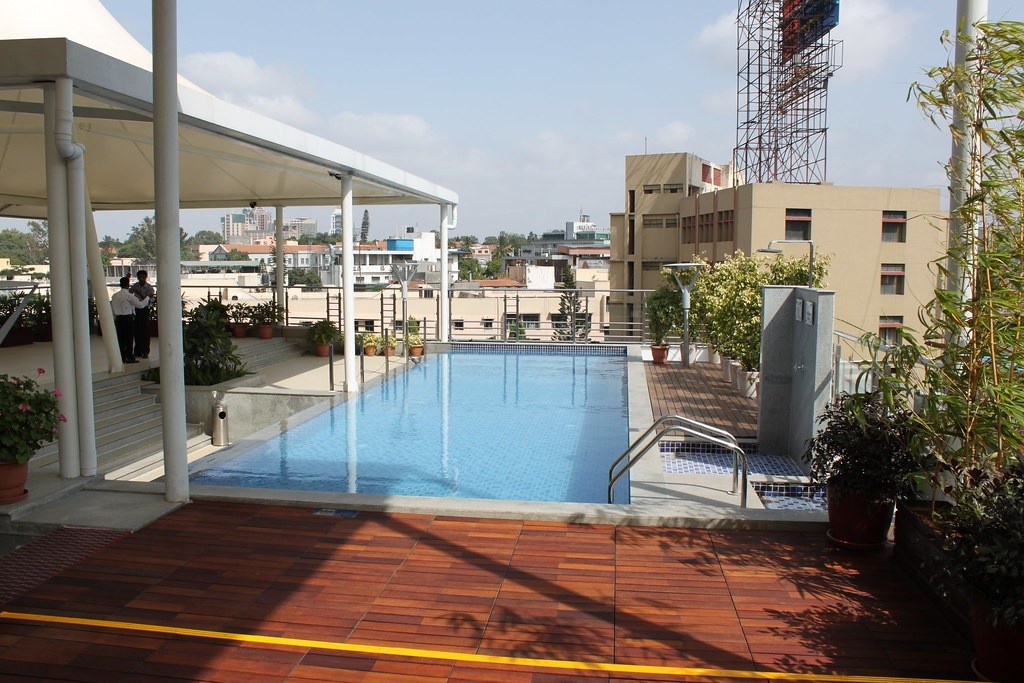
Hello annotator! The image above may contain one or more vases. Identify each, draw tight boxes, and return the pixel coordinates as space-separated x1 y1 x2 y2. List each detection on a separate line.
0 461 29 505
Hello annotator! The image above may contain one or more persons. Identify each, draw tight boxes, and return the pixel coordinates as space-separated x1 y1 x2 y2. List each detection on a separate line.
111 276 153 363
126 269 154 359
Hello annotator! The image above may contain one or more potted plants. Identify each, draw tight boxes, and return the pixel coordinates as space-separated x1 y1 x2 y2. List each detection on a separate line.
706 261 724 364
659 248 713 366
357 330 379 356
338 333 359 355
304 319 340 357
728 251 775 390
0 292 34 348
734 254 791 398
149 296 159 337
225 303 254 339
379 335 397 356
918 518 1024 683
250 300 285 339
403 332 424 356
712 249 751 383
799 389 952 552
643 286 683 363
28 292 52 342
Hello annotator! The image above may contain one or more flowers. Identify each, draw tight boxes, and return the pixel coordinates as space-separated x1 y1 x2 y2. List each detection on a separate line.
0 367 67 465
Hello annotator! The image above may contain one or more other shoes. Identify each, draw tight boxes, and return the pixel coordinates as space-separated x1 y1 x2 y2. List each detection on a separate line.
124 357 140 363
134 352 141 356
143 353 148 358
